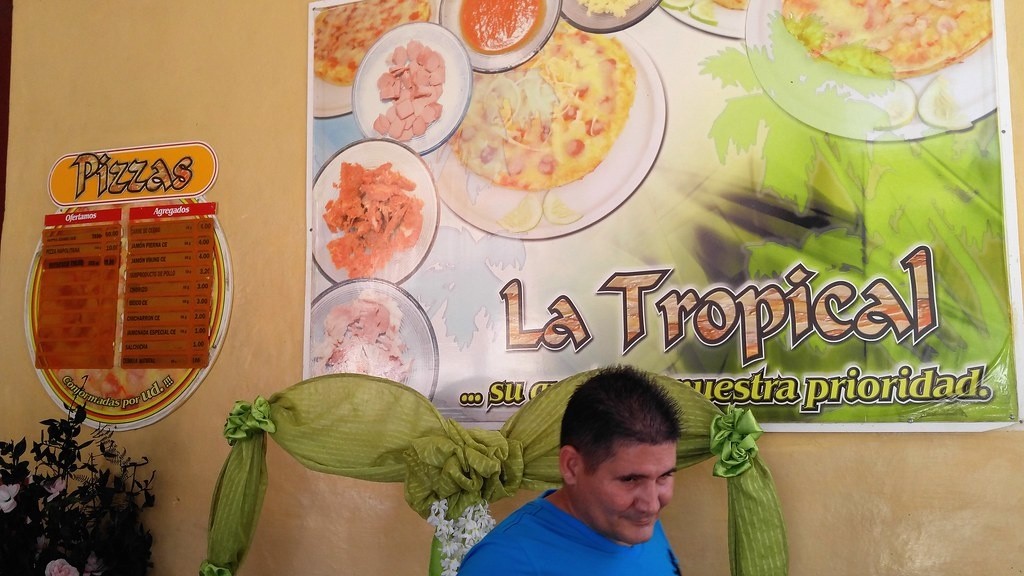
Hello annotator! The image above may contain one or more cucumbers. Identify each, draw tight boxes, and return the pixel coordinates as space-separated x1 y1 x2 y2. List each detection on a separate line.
543 190 583 224
690 0 717 26
660 0 694 10
497 191 542 232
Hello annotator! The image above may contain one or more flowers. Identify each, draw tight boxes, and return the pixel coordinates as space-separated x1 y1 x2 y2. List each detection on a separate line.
0 400 159 576
428 499 496 576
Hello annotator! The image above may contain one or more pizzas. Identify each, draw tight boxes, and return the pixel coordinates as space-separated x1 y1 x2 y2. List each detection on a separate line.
315 0 430 86
782 1 992 78
449 19 636 191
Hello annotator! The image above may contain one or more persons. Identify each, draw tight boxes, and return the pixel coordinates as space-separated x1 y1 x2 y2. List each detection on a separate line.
455 362 682 576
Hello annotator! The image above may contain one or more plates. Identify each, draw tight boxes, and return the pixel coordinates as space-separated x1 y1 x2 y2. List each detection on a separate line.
311 137 440 285
658 0 749 38
350 21 474 155
313 0 441 118
418 17 668 240
311 277 440 403
439 0 563 74
557 0 662 34
745 0 997 141
